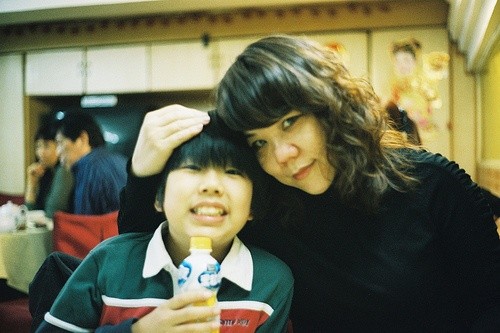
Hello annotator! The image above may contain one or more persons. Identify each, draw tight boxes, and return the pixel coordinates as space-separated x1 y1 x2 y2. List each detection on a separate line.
26 110 127 217
117 36 500 333
35 111 295 333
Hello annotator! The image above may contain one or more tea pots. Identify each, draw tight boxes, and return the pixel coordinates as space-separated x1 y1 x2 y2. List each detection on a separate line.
0 201 29 233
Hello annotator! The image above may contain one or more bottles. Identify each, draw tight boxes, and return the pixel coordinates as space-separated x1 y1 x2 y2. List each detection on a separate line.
177 237 222 333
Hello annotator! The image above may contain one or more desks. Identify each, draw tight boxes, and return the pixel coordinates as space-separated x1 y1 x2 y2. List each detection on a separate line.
0 222 54 295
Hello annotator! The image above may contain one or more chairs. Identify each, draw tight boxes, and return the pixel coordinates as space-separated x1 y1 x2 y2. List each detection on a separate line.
52 209 121 259
